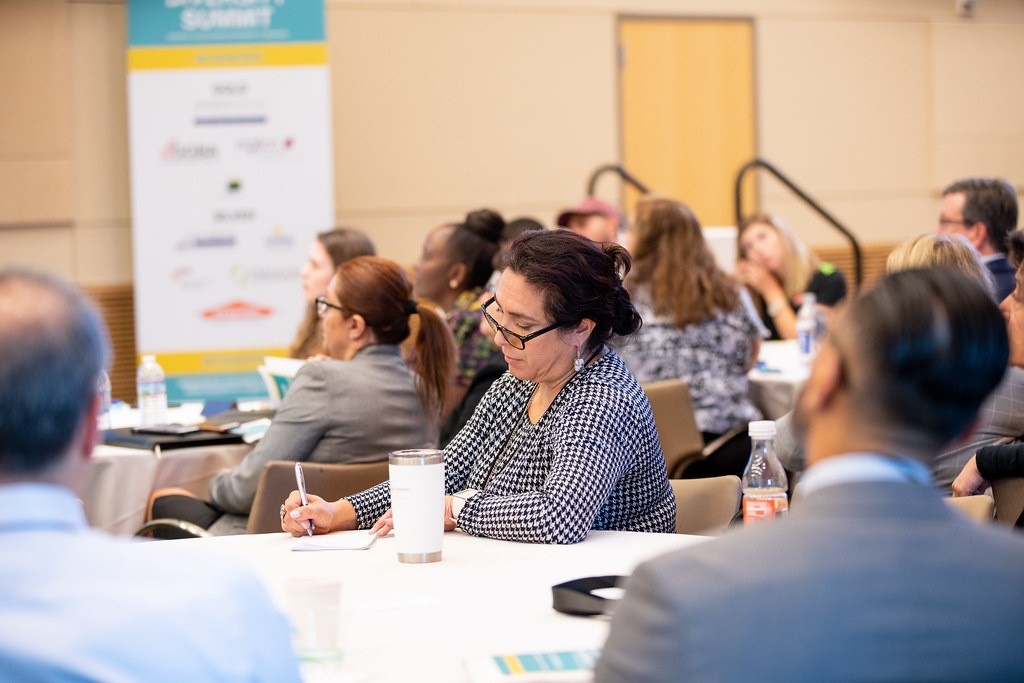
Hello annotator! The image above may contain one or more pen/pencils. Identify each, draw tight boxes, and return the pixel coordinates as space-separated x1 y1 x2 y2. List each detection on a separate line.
295 461 314 537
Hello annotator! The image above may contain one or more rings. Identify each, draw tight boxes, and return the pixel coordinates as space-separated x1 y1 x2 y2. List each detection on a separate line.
280 504 286 515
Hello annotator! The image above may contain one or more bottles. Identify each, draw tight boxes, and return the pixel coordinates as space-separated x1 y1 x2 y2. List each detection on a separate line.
796 291 819 358
739 419 790 526
132 348 169 426
88 362 114 419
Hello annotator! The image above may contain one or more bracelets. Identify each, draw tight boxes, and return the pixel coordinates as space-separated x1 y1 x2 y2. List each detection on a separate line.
767 298 786 316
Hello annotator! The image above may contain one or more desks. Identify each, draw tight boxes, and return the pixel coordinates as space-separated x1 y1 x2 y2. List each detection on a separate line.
745 340 811 420
84 426 255 538
153 530 724 683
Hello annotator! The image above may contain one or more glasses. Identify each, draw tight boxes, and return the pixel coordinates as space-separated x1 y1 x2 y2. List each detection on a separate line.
480 296 562 351
935 211 968 230
314 295 372 331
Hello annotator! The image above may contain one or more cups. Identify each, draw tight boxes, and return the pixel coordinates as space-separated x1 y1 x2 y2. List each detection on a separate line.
385 444 451 566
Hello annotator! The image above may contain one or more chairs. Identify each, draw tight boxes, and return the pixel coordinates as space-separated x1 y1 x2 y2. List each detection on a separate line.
640 378 705 474
669 473 743 538
248 461 388 534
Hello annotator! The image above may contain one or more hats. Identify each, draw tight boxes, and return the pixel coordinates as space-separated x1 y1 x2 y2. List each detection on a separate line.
556 200 621 239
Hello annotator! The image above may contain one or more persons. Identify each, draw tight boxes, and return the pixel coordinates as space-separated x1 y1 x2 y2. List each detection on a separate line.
145 255 452 540
291 229 377 360
0 270 298 683
769 176 1024 530
592 266 1024 683
732 213 847 341
281 227 676 544
402 197 795 525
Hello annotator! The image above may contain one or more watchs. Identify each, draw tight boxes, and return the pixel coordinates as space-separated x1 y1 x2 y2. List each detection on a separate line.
450 488 481 524
